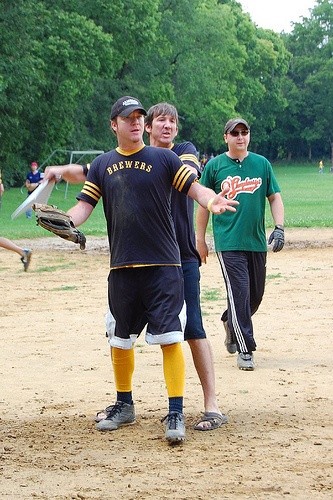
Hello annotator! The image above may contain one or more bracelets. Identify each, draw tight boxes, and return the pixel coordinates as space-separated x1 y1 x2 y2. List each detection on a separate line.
206 196 216 211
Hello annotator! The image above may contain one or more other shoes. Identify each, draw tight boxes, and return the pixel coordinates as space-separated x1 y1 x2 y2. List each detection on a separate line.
237 353 254 371
21 249 34 271
223 320 237 354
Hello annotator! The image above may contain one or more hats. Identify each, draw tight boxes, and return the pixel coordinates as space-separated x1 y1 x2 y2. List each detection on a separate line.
110 96 150 120
224 117 252 134
32 162 38 166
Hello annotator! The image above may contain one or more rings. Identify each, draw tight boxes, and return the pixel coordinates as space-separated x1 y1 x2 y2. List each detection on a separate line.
59 175 62 180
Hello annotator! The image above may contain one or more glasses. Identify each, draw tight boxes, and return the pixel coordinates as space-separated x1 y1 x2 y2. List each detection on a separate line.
225 130 250 136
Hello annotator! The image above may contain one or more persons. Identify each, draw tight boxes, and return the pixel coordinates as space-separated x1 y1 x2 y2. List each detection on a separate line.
33 95 242 443
199 118 285 372
319 159 324 175
197 151 222 171
0 237 33 272
42 102 229 431
25 161 44 218
0 178 6 209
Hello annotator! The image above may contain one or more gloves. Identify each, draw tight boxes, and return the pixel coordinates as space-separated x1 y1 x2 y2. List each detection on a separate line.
268 224 290 254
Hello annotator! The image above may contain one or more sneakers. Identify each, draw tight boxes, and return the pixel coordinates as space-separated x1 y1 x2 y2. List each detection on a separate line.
160 411 191 445
94 401 136 431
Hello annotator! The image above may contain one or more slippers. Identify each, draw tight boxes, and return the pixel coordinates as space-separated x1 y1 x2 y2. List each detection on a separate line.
193 413 226 431
96 404 115 422
